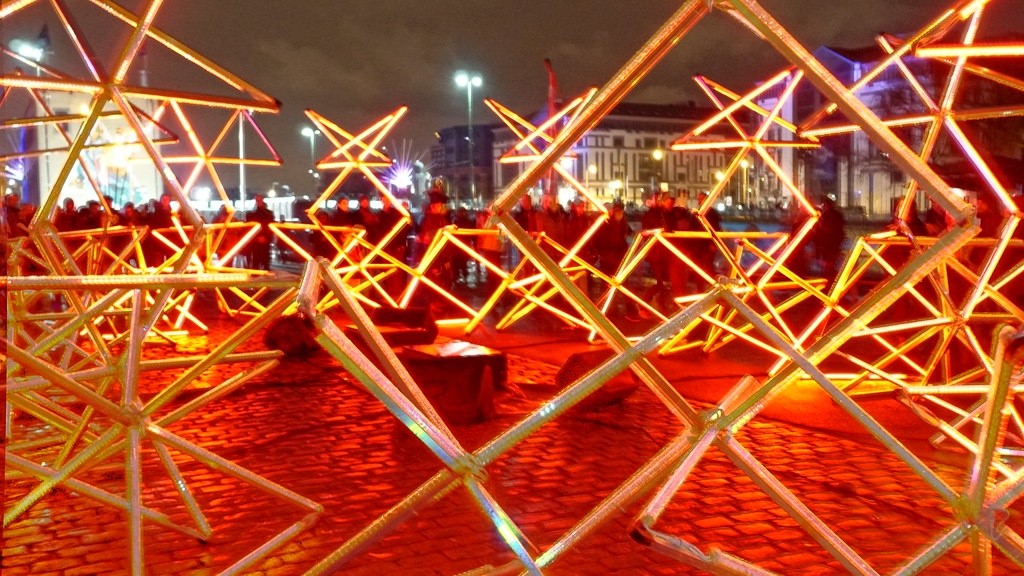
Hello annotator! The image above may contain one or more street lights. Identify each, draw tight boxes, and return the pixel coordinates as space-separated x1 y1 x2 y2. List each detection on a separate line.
650 151 664 191
456 71 483 203
585 165 596 190
301 127 322 200
742 160 749 205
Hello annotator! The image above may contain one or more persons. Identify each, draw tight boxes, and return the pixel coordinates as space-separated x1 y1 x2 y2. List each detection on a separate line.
195 194 286 270
815 193 847 279
882 195 1024 241
0 192 175 267
317 186 726 300
